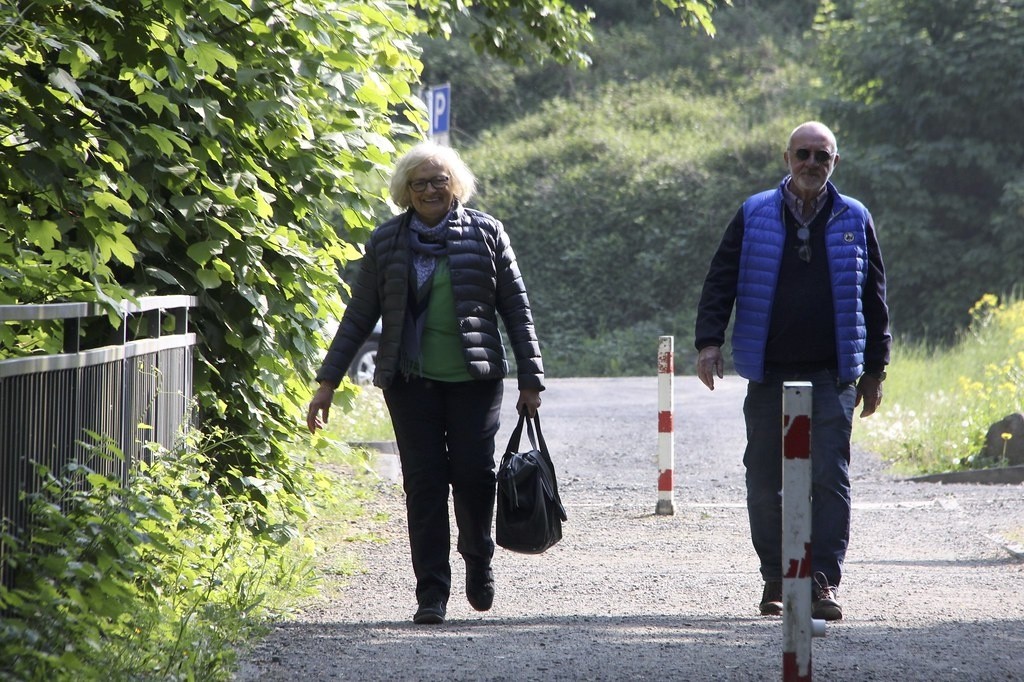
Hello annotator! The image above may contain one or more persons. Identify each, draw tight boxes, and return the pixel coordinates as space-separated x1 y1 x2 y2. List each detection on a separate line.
306 141 547 623
694 120 892 618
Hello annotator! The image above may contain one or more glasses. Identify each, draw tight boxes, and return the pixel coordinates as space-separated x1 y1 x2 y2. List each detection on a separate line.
787 148 835 163
407 175 450 192
797 223 812 263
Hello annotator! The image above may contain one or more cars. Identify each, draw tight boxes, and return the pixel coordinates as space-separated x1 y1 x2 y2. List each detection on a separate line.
346 316 384 388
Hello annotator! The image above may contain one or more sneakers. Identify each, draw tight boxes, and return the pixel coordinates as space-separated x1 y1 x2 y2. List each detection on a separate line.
812 571 842 621
414 598 446 623
466 560 494 611
759 581 783 616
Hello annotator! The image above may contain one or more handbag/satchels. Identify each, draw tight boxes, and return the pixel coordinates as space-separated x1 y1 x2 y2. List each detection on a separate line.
496 404 567 554
